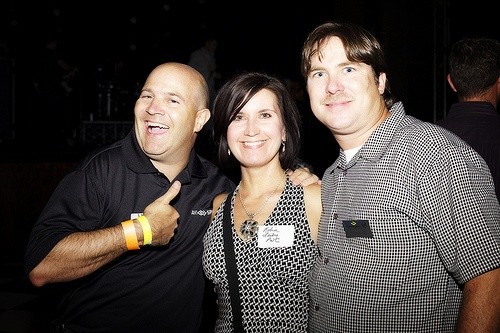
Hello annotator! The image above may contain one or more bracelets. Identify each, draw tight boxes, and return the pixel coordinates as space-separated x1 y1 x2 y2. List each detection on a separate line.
120 215 153 251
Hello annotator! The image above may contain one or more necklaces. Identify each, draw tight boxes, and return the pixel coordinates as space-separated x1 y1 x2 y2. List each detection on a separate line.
238 168 285 237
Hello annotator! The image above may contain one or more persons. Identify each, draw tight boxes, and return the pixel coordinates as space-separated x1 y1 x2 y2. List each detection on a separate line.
202 73 323 333
301 22 500 333
441 34 500 203
189 39 221 129
23 62 321 333
